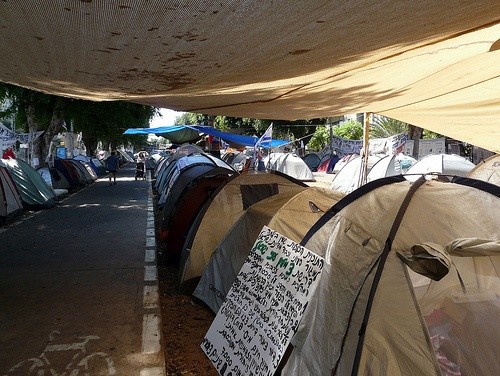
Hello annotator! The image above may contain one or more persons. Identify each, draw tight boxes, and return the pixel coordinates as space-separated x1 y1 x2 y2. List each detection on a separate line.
3 146 16 159
105 152 120 184
135 152 146 180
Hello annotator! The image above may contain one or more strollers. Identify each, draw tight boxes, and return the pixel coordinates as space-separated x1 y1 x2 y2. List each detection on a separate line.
135 163 146 181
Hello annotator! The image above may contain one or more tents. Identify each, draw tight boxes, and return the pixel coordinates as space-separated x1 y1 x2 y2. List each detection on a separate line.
0 151 133 227
122 123 500 376
